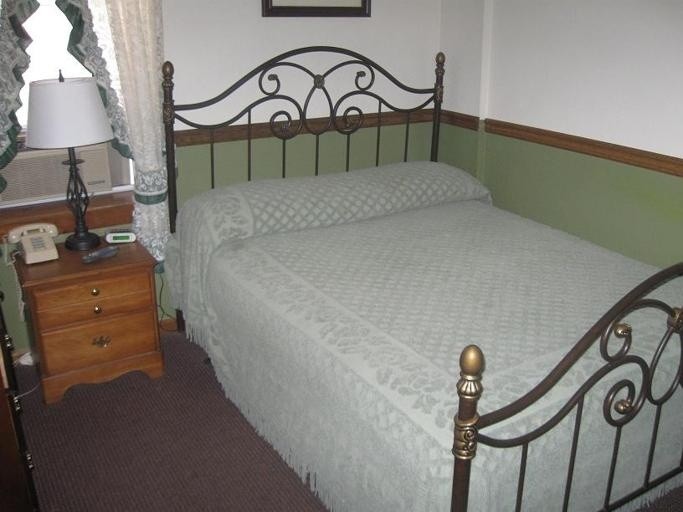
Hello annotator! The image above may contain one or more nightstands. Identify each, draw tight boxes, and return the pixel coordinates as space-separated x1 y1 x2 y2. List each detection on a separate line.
10 235 164 405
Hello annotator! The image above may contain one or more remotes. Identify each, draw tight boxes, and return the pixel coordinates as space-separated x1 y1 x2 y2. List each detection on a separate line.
82 244 120 264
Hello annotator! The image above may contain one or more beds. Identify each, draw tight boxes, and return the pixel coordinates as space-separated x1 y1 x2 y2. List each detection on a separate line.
160 44 683 511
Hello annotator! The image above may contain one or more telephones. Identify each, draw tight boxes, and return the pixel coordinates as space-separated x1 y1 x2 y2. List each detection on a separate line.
8 223 59 265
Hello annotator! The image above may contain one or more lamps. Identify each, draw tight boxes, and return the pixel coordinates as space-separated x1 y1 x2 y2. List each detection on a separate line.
23 70 114 250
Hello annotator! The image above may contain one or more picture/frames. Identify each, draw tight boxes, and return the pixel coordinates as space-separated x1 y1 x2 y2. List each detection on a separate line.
261 0 371 18
